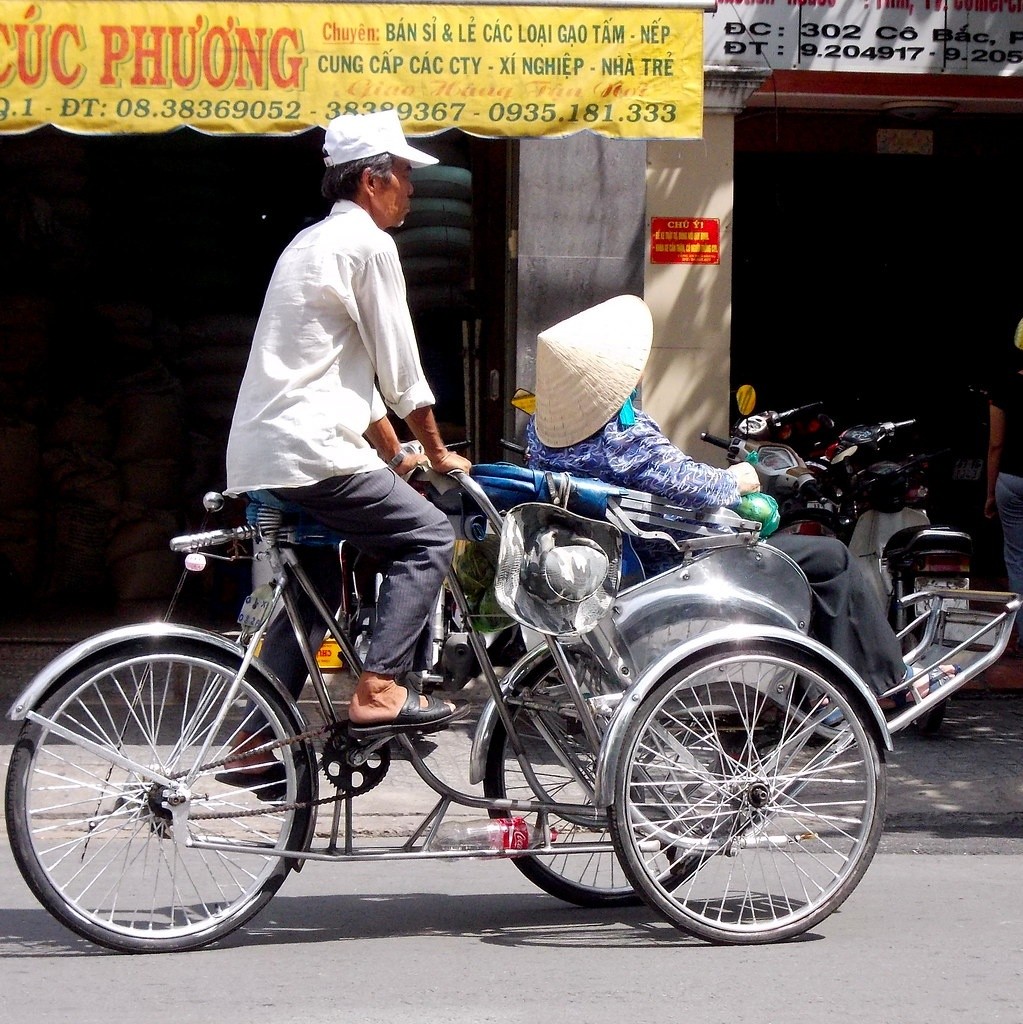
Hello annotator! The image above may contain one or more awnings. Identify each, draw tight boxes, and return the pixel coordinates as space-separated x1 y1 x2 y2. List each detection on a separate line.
0 1 715 143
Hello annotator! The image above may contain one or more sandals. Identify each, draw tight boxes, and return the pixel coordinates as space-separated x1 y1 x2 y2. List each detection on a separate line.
812 692 844 726
882 664 962 716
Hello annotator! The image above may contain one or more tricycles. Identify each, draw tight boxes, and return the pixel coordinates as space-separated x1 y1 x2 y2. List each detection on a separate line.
2 440 1020 954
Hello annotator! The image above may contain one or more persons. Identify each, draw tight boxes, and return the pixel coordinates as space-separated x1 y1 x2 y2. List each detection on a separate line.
526 293 961 726
216 110 470 794
983 319 1023 643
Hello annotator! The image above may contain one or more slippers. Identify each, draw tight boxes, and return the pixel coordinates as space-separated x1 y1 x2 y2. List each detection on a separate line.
215 759 288 800
347 685 472 739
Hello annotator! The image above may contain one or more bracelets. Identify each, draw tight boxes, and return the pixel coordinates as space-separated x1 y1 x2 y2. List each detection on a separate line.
387 447 411 470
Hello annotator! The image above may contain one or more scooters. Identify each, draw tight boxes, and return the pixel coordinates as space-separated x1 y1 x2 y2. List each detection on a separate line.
701 397 981 739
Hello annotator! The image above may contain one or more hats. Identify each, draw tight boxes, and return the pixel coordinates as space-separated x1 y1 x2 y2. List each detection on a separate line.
537 294 654 448
323 109 440 169
